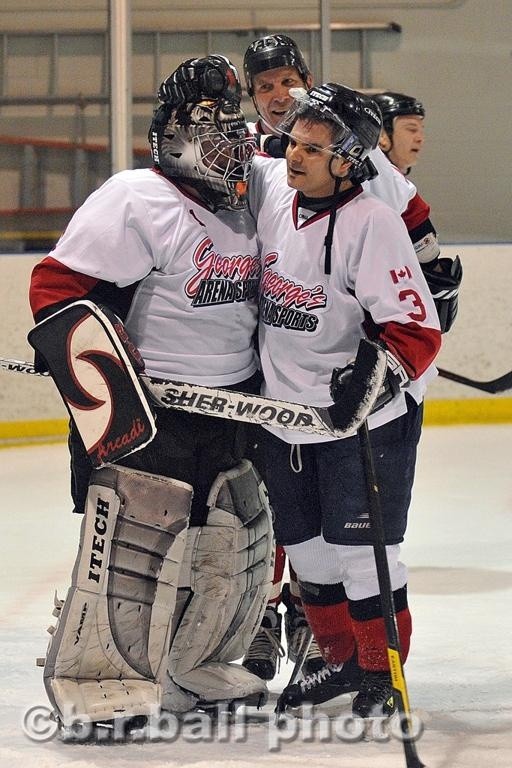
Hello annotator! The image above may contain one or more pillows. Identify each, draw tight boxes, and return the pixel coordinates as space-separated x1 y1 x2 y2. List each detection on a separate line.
244 35 309 82
147 86 425 199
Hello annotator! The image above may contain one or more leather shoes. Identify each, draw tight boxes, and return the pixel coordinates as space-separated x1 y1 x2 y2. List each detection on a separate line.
334 345 411 419
420 252 462 335
160 55 240 113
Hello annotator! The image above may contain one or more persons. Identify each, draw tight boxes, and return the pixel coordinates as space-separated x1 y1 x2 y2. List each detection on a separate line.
26 50 263 527
238 33 464 680
370 90 428 176
233 76 446 723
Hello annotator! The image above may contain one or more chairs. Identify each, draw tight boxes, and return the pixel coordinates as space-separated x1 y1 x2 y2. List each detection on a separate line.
248 608 400 718
